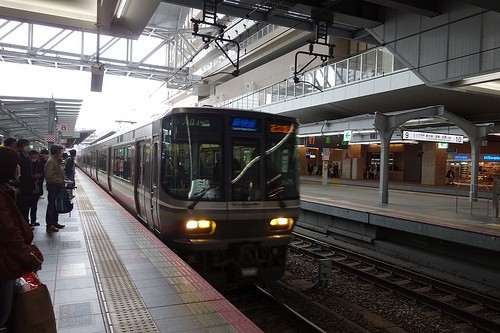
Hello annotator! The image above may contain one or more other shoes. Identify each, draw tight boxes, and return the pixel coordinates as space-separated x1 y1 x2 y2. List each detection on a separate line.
46 224 58 232
54 223 65 228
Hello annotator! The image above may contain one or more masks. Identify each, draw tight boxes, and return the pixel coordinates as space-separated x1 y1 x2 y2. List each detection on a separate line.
31 158 37 162
24 146 30 152
14 164 21 180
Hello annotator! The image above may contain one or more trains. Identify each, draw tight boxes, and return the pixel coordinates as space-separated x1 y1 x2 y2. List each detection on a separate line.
74 107 300 261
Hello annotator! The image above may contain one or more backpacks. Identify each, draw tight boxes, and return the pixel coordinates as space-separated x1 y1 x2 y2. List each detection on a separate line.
54 186 74 213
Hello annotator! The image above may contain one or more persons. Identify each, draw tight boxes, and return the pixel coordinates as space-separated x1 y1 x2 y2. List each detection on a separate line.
28 150 44 226
3 137 17 153
39 148 459 198
0 146 42 333
493 174 500 218
17 139 35 224
43 146 65 233
